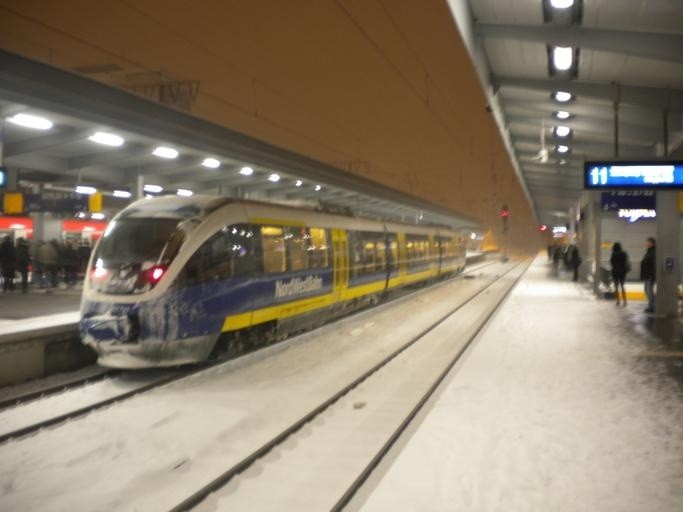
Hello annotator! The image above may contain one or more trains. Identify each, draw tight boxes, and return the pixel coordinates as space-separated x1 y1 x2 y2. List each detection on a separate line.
76 195 466 372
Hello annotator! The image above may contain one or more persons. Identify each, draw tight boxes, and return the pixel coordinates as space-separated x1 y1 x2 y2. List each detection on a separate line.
545 236 583 281
608 241 629 308
0 233 95 294
638 237 658 313
